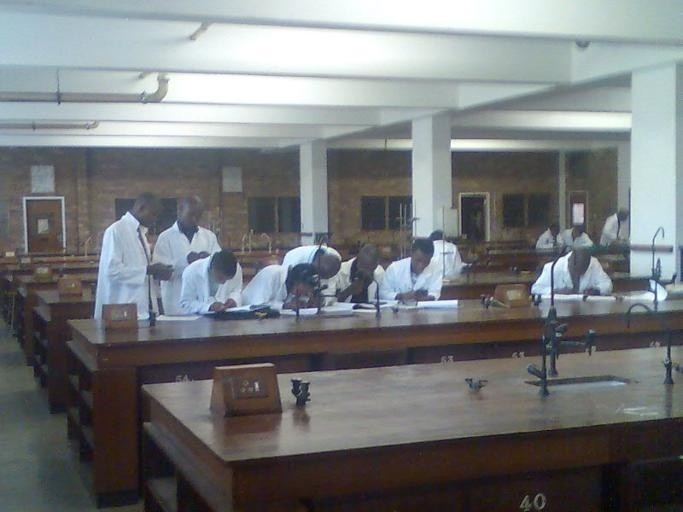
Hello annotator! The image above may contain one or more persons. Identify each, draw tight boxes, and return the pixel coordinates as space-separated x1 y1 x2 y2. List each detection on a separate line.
380 239 443 303
336 242 384 303
560 225 593 251
240 263 322 314
531 247 613 293
153 193 219 316
600 207 629 249
94 191 174 318
284 245 341 307
429 230 461 280
181 249 242 314
534 223 563 249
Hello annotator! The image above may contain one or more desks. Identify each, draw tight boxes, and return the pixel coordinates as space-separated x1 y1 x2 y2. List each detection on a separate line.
0 244 683 511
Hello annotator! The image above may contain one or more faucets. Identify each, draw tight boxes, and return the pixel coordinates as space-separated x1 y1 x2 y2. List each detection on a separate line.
526 316 569 394
241 228 679 311
624 300 677 385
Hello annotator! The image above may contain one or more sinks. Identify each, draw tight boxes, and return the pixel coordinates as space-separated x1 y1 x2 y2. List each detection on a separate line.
526 375 631 393
